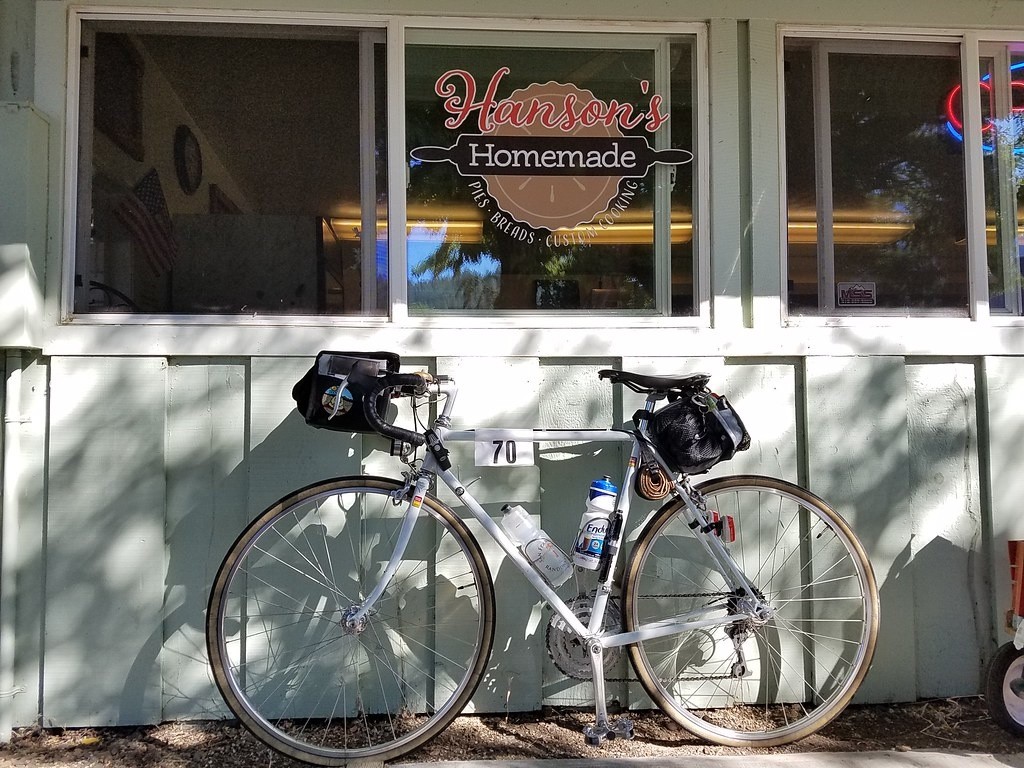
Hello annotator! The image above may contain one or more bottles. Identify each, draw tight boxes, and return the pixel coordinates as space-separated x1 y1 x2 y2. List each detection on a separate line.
501 504 574 587
570 475 619 571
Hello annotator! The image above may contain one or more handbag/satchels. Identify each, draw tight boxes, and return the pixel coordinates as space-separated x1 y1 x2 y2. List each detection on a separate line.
292 351 400 432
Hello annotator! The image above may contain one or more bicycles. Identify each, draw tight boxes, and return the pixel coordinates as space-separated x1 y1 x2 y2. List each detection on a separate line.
205 367 883 767
